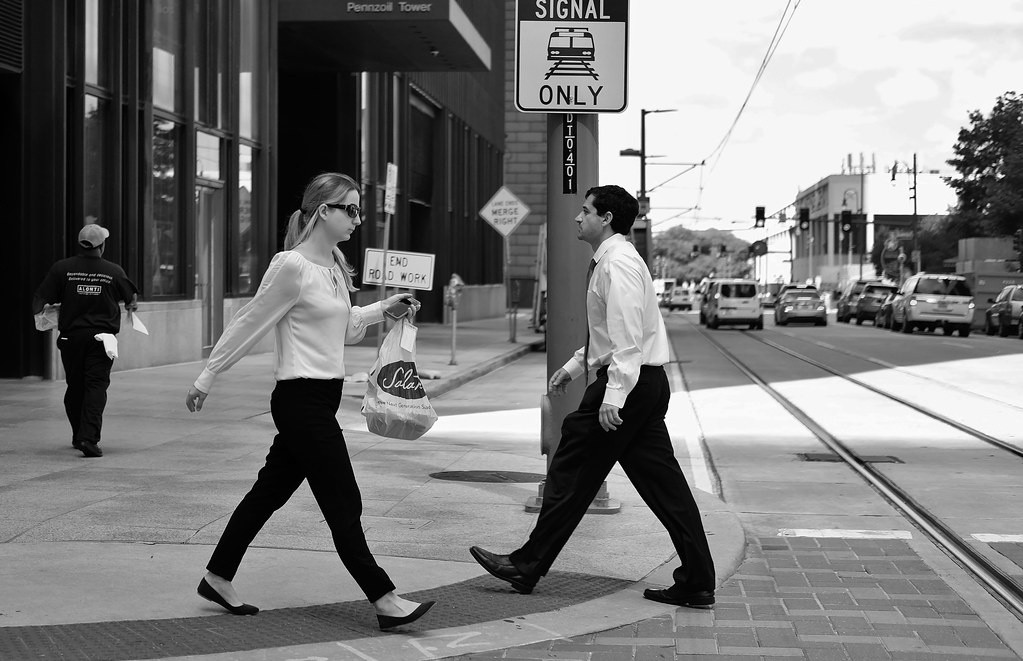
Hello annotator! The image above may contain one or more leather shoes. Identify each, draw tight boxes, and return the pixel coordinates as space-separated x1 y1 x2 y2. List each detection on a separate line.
469 545 541 595
643 583 715 606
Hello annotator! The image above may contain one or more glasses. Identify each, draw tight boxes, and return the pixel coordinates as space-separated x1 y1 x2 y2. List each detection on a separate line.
316 203 363 219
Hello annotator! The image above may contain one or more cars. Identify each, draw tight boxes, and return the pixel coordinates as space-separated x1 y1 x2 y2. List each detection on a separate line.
656 290 671 306
875 289 895 329
773 284 827 327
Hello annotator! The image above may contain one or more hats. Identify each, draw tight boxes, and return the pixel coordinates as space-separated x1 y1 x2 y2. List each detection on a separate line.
78 223 110 249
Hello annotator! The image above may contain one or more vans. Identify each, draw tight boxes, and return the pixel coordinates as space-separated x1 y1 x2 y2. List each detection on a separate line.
696 278 763 331
668 287 693 311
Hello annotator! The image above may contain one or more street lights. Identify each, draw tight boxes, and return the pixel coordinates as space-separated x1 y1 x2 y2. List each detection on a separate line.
891 160 920 273
842 188 862 282
619 109 675 218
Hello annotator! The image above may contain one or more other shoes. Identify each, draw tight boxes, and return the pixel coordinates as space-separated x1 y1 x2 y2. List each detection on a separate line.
376 602 437 630
197 578 260 616
71 437 103 458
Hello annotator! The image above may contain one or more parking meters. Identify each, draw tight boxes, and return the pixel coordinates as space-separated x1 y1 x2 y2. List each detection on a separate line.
446 277 463 363
511 281 520 343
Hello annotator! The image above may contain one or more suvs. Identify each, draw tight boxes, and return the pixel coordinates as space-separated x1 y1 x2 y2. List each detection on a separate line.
891 275 975 337
854 282 892 325
984 285 1023 336
834 283 869 322
758 285 783 306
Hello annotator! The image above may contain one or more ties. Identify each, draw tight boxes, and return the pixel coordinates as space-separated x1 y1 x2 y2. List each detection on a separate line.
583 259 597 388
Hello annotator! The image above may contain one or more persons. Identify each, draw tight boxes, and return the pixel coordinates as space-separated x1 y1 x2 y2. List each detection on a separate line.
42 223 138 457
185 174 437 630
468 185 716 606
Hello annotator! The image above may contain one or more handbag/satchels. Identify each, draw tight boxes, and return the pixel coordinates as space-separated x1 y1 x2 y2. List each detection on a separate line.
33 304 59 331
362 316 439 441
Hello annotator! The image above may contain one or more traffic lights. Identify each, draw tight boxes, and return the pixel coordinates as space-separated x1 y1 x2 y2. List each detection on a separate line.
755 207 765 228
799 208 810 229
841 211 852 232
